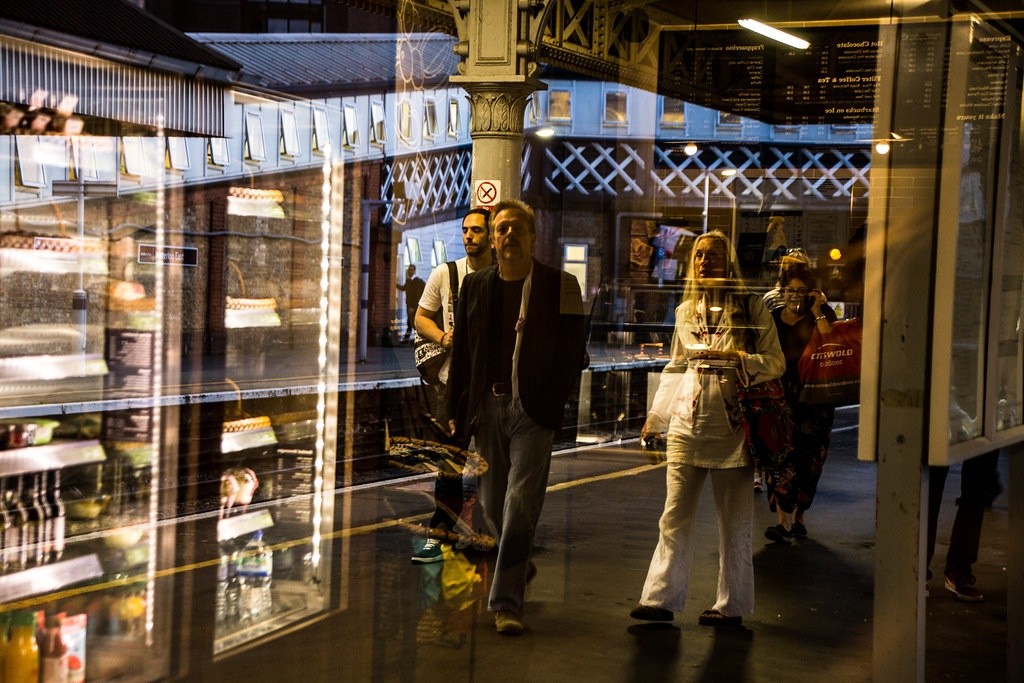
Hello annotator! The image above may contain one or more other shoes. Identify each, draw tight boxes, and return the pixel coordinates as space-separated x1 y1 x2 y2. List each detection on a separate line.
698 610 743 625
629 607 673 622
753 477 763 492
765 525 793 541
788 522 808 536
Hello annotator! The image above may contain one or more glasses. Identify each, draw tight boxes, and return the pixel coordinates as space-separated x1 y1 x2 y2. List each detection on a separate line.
785 288 807 297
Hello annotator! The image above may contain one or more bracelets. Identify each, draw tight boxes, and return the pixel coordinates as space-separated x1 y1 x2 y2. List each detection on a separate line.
816 315 826 321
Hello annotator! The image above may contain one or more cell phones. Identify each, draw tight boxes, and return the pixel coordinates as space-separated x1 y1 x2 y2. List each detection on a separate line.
802 293 814 310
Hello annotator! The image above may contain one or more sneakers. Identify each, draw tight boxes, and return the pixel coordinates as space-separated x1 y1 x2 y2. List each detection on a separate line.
411 538 456 562
944 573 985 601
926 578 930 597
527 562 537 583
495 610 524 633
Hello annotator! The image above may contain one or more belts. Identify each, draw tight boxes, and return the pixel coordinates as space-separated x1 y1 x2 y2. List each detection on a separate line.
486 382 509 396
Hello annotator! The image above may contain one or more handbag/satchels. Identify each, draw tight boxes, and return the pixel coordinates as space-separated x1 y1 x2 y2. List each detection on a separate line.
735 377 794 470
797 317 861 408
413 261 459 401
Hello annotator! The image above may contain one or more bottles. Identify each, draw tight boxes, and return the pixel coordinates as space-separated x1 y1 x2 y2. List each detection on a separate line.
215 530 274 622
0 609 86 683
0 469 66 575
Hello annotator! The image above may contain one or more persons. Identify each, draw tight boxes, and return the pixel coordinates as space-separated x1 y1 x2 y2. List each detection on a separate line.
752 248 999 603
630 231 786 623
386 201 589 632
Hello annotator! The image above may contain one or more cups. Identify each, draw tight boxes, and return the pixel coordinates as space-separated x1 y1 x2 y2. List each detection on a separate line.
684 344 710 363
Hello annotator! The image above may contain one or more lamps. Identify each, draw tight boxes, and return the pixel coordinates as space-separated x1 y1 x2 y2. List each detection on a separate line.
738 14 812 50
